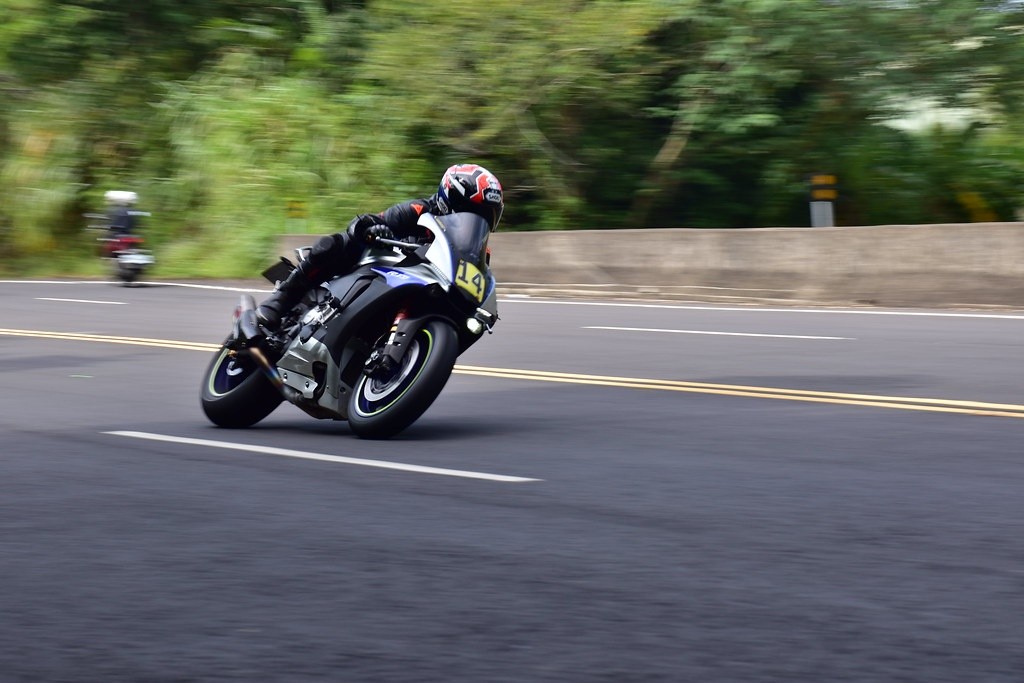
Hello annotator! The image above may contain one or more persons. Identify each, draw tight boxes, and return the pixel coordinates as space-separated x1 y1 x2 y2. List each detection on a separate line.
251 163 505 332
96 192 139 254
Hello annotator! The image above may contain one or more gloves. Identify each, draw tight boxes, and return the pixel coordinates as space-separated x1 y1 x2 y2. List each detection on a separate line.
364 224 394 252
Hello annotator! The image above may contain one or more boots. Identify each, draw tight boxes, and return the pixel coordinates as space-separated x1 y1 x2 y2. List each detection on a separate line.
255 259 315 330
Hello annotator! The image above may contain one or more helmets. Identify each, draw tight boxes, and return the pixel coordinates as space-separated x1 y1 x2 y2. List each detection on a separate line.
436 164 504 233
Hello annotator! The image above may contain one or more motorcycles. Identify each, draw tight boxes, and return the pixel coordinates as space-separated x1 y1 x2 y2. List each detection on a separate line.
198 208 502 439
103 229 154 280
